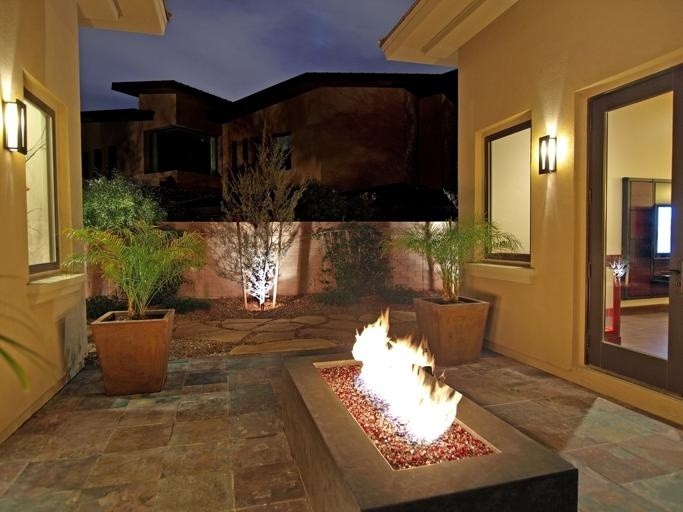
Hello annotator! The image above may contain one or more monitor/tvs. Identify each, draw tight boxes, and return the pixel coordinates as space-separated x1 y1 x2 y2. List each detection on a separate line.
653 204 672 259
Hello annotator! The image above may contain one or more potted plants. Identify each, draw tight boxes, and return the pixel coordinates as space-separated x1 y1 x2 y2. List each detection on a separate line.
57 168 209 396
392 215 521 368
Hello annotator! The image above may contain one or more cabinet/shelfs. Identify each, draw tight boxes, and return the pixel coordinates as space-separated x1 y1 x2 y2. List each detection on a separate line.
622 176 672 299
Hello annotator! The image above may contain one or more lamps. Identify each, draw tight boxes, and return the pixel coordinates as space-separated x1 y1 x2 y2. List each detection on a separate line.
2 99 28 155
538 134 557 174
